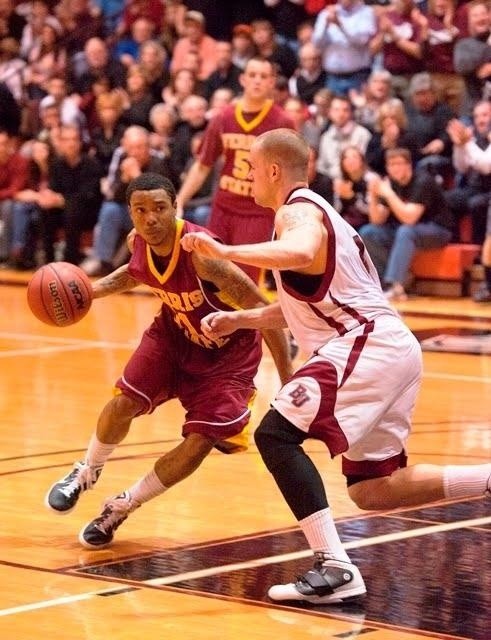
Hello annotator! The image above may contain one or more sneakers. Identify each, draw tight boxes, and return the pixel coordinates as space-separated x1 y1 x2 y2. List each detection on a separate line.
46 460 101 511
77 492 139 549
381 283 407 301
267 553 367 605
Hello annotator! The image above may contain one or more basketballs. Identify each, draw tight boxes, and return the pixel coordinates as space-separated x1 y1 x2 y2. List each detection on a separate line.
27 261 95 327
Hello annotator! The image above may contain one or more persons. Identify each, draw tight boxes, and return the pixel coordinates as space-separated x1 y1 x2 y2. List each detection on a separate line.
0 0 491 302
42 174 296 551
175 125 491 607
